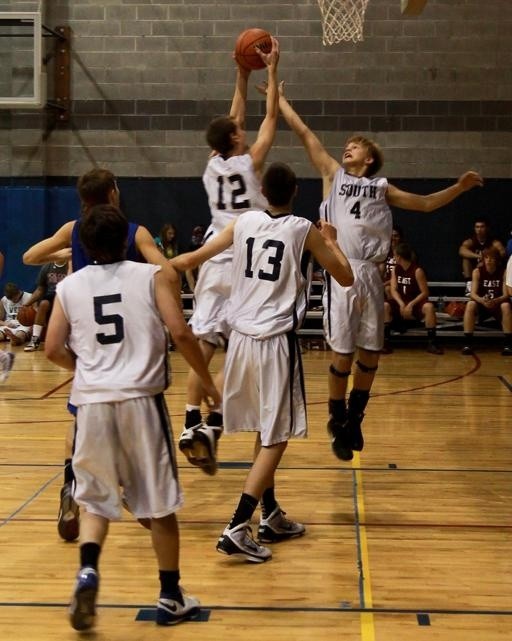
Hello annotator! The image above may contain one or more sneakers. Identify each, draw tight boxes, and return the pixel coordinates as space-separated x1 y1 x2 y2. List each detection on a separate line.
0 352 16 382
346 407 365 451
23 334 40 353
56 482 80 541
191 421 224 476
67 565 99 631
256 504 306 542
120 492 151 530
177 420 203 465
215 519 272 562
380 339 512 356
155 594 202 626
325 413 353 461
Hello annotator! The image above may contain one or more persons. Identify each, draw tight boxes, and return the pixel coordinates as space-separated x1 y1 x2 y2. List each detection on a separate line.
0 351 13 384
253 81 484 463
44 205 226 632
167 164 356 562
21 170 181 541
179 36 279 475
2 219 512 356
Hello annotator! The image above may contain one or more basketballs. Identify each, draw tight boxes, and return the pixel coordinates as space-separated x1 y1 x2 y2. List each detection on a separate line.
235 29 272 70
17 306 37 326
